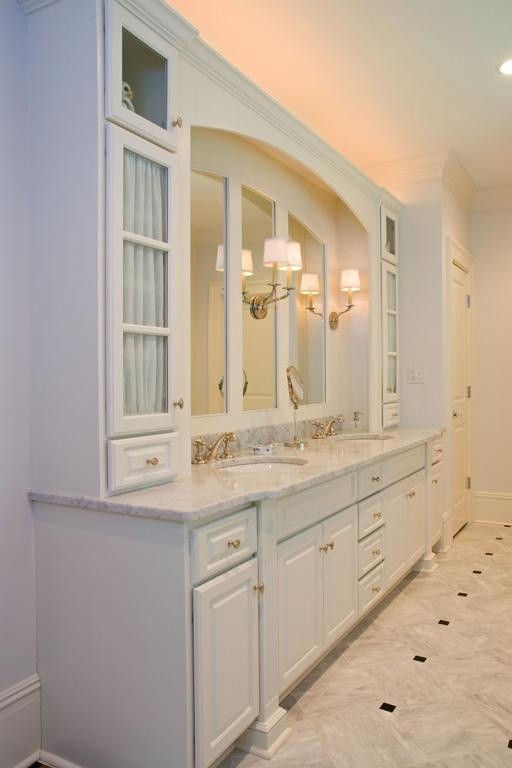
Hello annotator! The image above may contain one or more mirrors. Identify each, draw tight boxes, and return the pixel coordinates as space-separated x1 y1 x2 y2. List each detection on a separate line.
239 184 277 411
287 209 326 406
284 364 308 449
190 167 228 417
219 369 248 398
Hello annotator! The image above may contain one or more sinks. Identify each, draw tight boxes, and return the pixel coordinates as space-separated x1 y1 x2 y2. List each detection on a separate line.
330 434 395 441
211 456 308 472
321 417 344 438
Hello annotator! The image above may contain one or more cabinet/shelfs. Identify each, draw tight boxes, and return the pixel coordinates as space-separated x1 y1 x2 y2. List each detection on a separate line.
354 460 386 620
427 434 446 548
278 469 359 699
30 500 257 768
380 204 402 428
18 0 188 500
384 441 427 595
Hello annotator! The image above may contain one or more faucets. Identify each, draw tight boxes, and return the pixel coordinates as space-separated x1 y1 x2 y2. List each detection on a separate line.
204 432 238 461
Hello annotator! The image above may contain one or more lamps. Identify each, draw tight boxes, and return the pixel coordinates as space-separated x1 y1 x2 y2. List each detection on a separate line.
251 235 302 320
329 269 361 330
299 273 325 318
216 245 257 319
